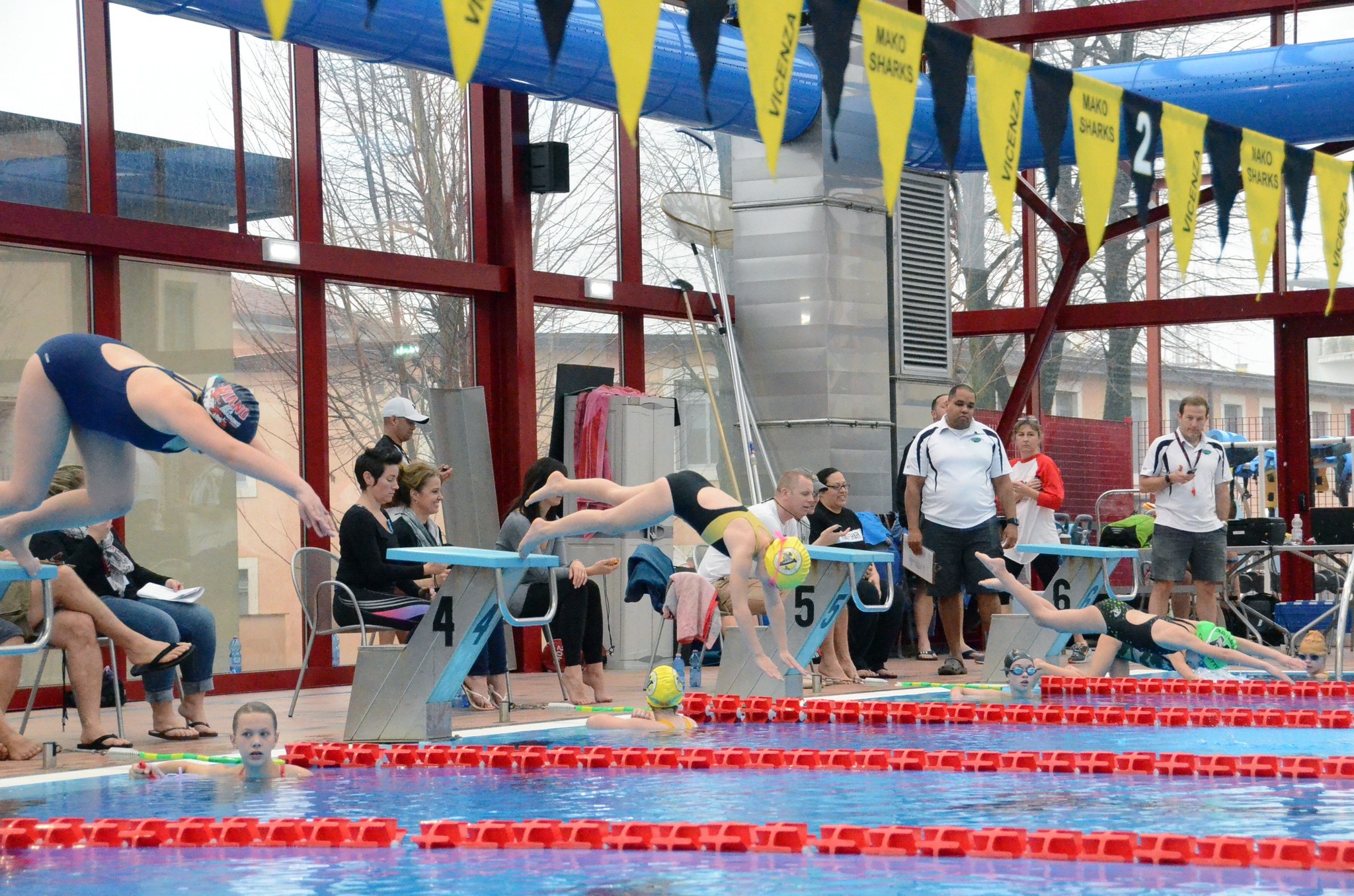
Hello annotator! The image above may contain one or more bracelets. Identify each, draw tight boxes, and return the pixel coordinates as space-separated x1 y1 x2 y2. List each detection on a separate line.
1165 474 1173 485
433 575 440 589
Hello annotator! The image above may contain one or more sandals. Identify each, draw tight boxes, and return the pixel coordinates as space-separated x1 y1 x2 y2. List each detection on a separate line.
938 657 968 675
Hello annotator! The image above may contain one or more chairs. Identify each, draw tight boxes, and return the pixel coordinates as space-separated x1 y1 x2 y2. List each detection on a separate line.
286 543 405 721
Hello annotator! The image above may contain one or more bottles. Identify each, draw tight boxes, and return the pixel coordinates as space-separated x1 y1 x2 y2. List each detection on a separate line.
229 635 242 673
1291 513 1303 544
673 654 685 691
332 633 340 667
689 649 701 687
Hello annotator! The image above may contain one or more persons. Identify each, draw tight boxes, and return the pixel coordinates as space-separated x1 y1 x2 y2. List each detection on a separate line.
371 400 453 522
1172 551 1239 619
0 333 339 576
588 666 699 730
518 469 810 680
903 386 1019 676
951 651 1040 700
795 469 864 682
0 617 43 760
974 549 1307 684
391 461 510 710
29 461 222 743
1000 416 1092 663
806 470 904 678
691 470 851 613
0 541 194 749
129 703 313 778
1297 629 1328 679
331 445 448 642
1032 633 1238 679
897 393 981 661
1139 398 1232 619
496 458 621 705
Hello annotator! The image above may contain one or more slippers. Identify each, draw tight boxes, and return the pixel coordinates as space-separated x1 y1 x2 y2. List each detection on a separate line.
463 680 514 710
857 667 898 679
962 648 986 659
915 648 938 661
130 642 196 677
148 722 217 739
77 733 133 751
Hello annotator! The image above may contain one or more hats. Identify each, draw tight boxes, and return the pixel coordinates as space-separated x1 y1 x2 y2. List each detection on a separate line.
1004 648 1034 676
382 397 430 425
790 467 829 492
1298 630 1327 655
765 535 812 590
1195 620 1240 671
198 376 259 444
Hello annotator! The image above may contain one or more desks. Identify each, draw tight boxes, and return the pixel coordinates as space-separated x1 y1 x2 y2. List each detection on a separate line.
1135 538 1352 662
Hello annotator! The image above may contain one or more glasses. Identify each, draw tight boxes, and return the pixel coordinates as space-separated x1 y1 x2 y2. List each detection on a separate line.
825 484 850 490
810 493 823 497
1006 667 1038 675
767 530 786 590
1226 559 1239 564
1299 653 1318 659
1198 653 1207 670
1017 416 1038 423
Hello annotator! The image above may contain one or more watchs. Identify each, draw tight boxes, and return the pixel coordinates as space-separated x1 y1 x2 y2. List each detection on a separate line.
1007 518 1019 526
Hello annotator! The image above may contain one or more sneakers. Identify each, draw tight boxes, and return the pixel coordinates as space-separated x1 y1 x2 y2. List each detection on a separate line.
1068 643 1092 663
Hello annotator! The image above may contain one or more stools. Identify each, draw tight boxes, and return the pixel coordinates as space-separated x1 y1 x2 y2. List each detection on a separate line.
28 632 184 749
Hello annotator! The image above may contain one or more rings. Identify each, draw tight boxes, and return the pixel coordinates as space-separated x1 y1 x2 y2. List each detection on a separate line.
1014 488 1016 492
